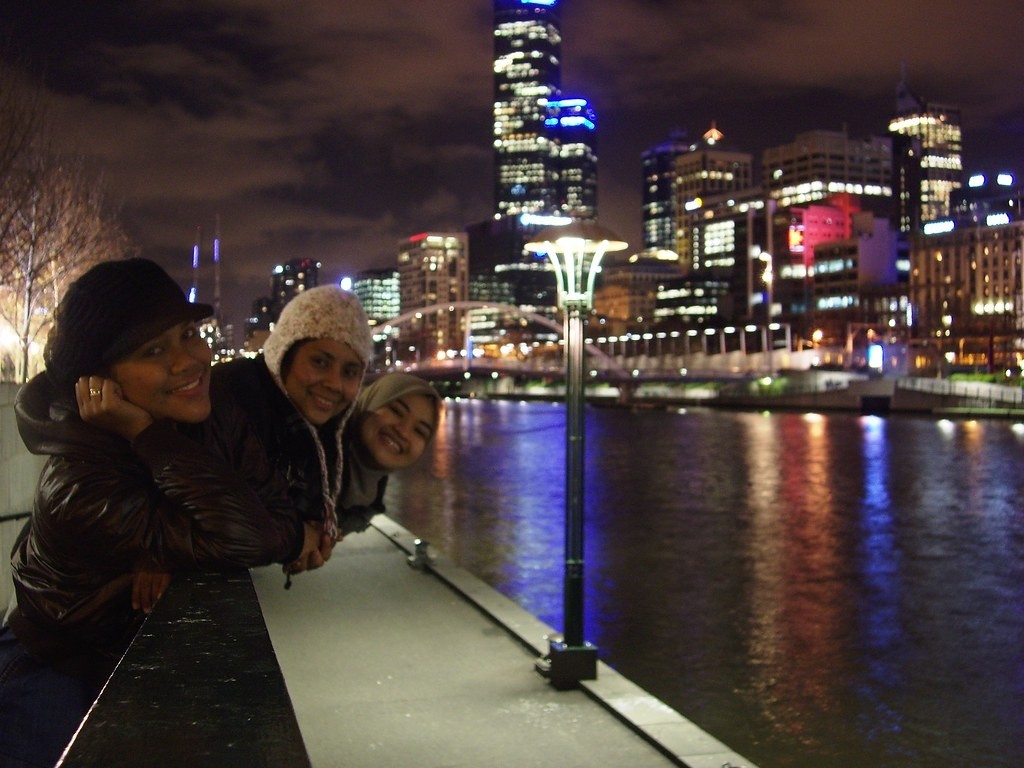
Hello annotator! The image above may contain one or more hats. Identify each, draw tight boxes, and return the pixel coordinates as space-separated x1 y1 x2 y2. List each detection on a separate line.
43 257 214 388
262 283 374 535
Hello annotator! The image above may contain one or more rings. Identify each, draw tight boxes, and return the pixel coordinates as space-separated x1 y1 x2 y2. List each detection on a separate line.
90 388 102 397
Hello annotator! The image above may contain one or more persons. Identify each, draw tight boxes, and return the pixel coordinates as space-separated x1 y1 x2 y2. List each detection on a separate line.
208 284 442 574
0 257 304 768
